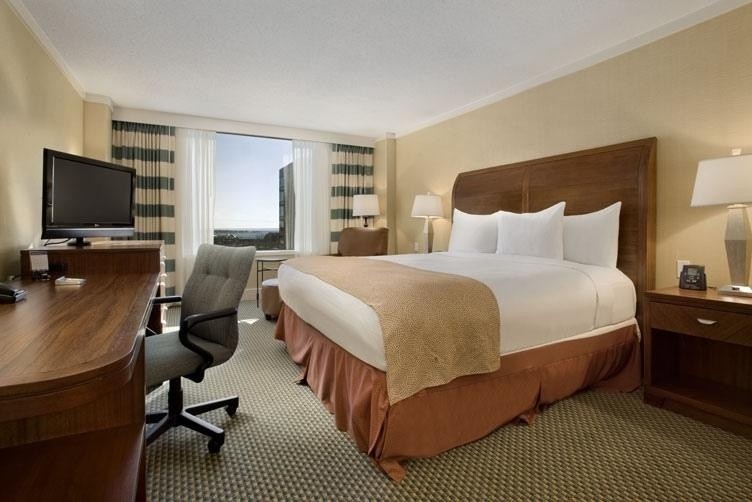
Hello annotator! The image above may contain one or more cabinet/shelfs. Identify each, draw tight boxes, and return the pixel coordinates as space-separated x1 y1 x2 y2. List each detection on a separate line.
18 240 168 396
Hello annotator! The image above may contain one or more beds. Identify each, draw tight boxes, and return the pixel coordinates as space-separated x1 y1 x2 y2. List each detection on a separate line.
273 134 659 484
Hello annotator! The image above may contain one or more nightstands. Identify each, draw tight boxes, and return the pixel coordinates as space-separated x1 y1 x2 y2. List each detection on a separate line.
641 281 752 442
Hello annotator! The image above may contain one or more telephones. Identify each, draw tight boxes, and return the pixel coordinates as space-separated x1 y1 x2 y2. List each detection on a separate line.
0 283 26 303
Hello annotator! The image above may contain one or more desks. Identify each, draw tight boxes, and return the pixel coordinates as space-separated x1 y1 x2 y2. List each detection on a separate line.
0 272 163 502
256 258 288 308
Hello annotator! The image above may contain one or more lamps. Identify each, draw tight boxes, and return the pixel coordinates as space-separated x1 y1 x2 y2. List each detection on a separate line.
352 194 381 228
690 146 752 287
410 191 444 253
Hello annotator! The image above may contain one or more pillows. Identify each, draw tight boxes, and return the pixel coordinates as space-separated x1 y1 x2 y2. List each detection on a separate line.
448 200 623 269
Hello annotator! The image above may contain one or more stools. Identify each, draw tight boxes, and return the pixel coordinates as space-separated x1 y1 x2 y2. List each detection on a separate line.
261 278 283 323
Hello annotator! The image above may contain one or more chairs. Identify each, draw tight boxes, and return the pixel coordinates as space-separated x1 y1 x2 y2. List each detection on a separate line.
338 227 389 257
143 242 257 456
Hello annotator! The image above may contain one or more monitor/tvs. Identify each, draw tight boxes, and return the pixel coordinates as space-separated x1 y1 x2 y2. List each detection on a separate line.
40 148 136 246
